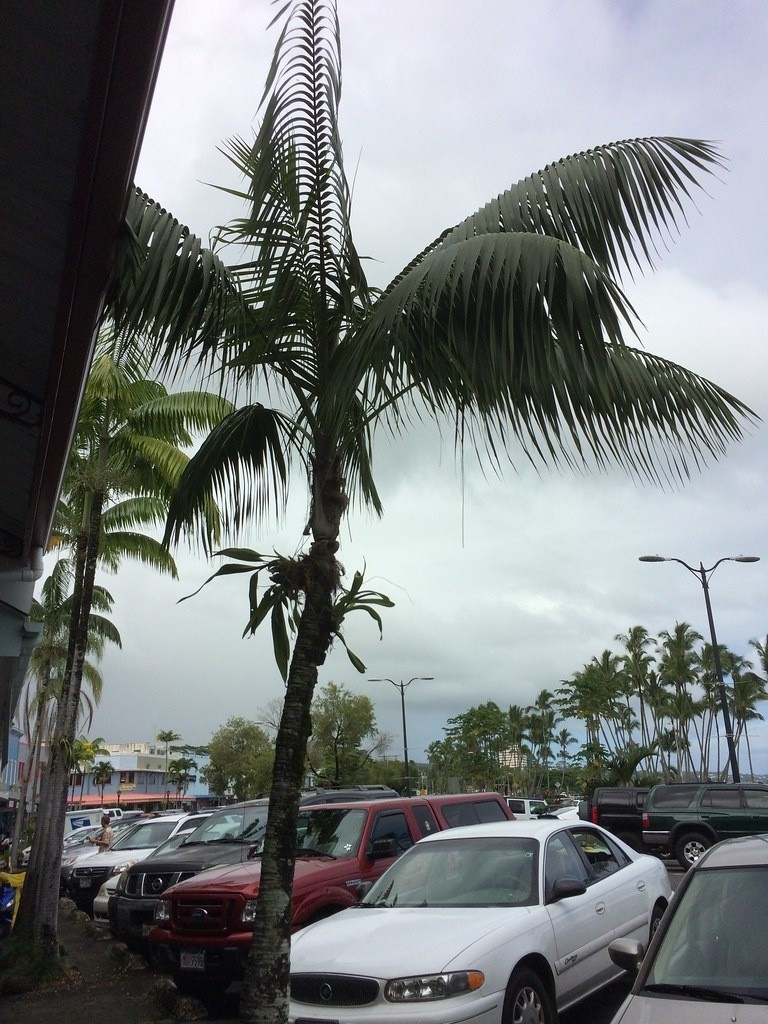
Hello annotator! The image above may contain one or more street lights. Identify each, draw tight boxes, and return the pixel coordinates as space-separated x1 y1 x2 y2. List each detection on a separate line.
638 553 761 783
116 790 122 807
367 677 435 797
166 790 171 808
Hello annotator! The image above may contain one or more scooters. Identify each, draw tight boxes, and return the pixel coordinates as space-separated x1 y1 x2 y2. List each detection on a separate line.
0 884 17 938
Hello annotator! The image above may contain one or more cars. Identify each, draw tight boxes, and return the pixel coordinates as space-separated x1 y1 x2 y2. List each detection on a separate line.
20 804 243 926
291 815 676 1023
501 789 582 822
609 833 768 1024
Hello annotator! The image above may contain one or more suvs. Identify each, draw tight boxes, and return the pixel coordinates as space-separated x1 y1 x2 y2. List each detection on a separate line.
107 784 409 973
577 786 675 860
137 789 526 996
641 782 768 873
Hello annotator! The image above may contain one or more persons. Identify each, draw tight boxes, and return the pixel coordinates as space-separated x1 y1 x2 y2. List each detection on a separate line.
89 815 113 853
